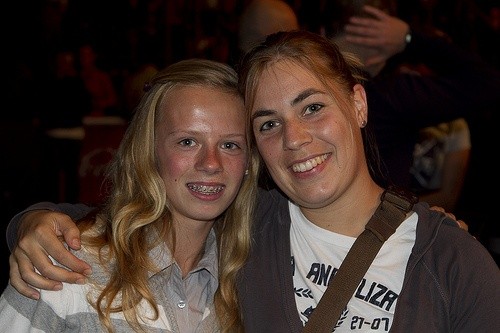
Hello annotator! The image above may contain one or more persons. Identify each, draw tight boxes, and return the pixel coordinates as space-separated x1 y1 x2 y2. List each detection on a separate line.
7 30 500 333
0 58 469 333
0 0 500 263
